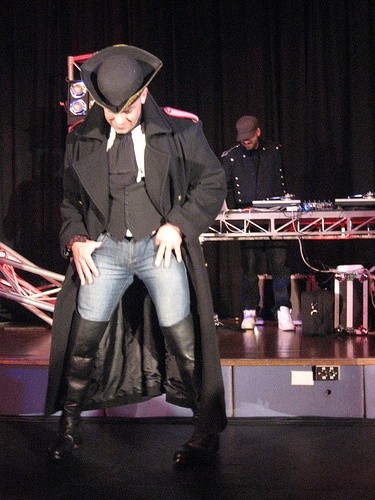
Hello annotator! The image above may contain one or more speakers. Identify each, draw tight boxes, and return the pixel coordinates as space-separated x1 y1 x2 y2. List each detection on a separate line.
301 292 340 337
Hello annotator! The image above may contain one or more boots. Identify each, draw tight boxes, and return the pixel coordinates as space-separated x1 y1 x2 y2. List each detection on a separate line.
160 313 220 465
49 311 109 461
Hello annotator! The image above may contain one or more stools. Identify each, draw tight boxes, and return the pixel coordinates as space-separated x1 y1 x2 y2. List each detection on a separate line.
257 273 316 326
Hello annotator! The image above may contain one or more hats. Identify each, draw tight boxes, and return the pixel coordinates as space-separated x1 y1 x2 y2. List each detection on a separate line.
235 116 259 140
79 46 163 113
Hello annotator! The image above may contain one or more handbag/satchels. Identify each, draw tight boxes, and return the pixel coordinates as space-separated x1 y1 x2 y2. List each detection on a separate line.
300 289 334 335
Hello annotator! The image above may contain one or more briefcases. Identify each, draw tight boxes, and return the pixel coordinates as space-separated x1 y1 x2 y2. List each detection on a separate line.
334 272 370 335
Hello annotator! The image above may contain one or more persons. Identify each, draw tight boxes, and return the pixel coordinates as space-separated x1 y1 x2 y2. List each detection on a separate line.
222 116 296 332
44 45 229 467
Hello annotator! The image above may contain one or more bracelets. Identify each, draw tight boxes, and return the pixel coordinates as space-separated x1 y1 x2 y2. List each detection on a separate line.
67 235 86 249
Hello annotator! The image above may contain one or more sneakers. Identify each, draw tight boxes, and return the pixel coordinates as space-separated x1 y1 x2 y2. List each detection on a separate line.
277 305 295 330
241 308 256 330
